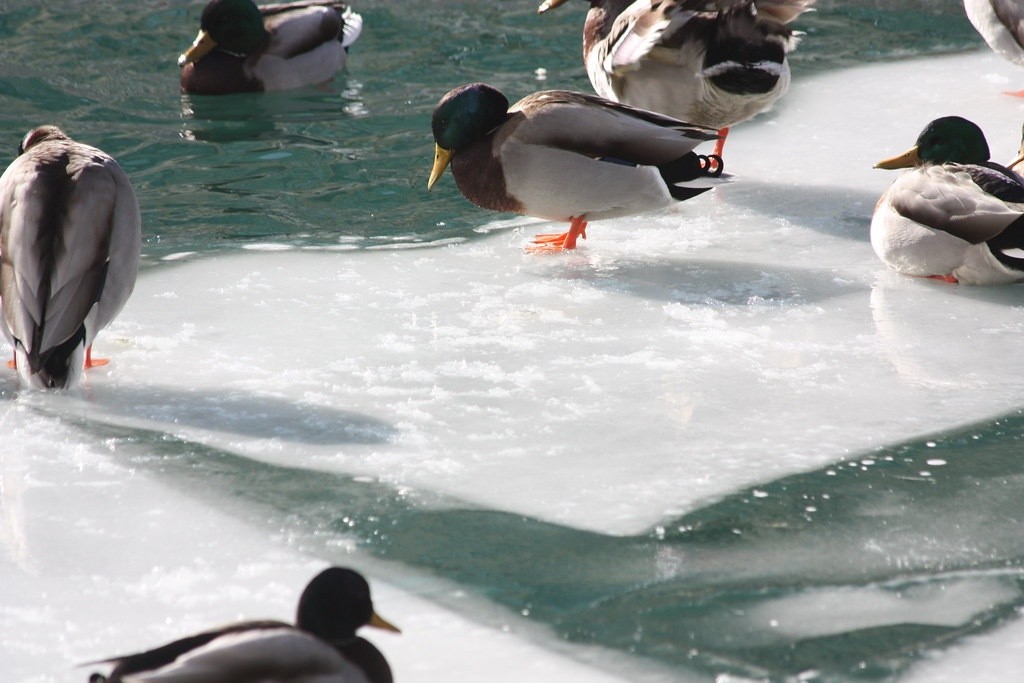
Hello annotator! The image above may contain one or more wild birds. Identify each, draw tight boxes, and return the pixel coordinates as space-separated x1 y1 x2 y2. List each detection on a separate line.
0 124 141 394
70 563 402 683
176 0 362 100
427 0 819 257
868 0 1024 286
